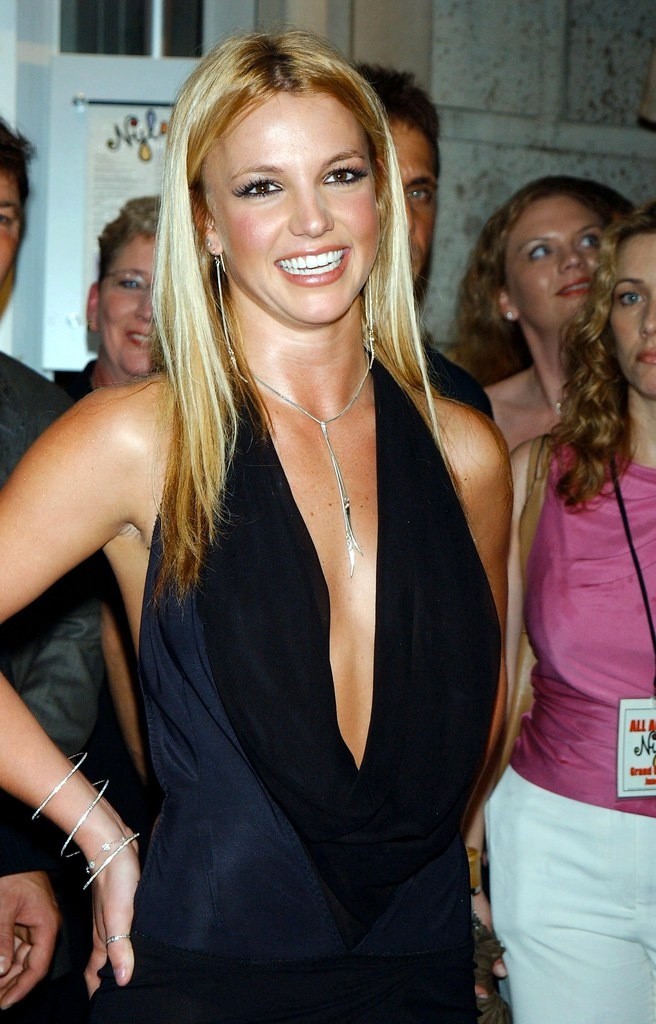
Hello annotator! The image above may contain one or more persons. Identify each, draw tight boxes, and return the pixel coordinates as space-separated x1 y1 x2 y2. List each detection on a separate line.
0 25 517 1024
453 174 656 1024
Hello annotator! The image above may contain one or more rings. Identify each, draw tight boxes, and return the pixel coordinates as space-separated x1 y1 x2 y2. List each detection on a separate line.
106 934 130 944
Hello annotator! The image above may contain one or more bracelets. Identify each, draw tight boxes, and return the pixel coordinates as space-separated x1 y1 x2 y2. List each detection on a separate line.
33 750 141 887
471 886 481 896
86 835 130 872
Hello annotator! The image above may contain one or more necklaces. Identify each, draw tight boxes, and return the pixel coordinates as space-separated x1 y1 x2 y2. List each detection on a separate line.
244 351 371 578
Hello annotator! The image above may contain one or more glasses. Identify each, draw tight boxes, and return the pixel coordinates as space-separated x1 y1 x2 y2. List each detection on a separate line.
99 269 151 297
